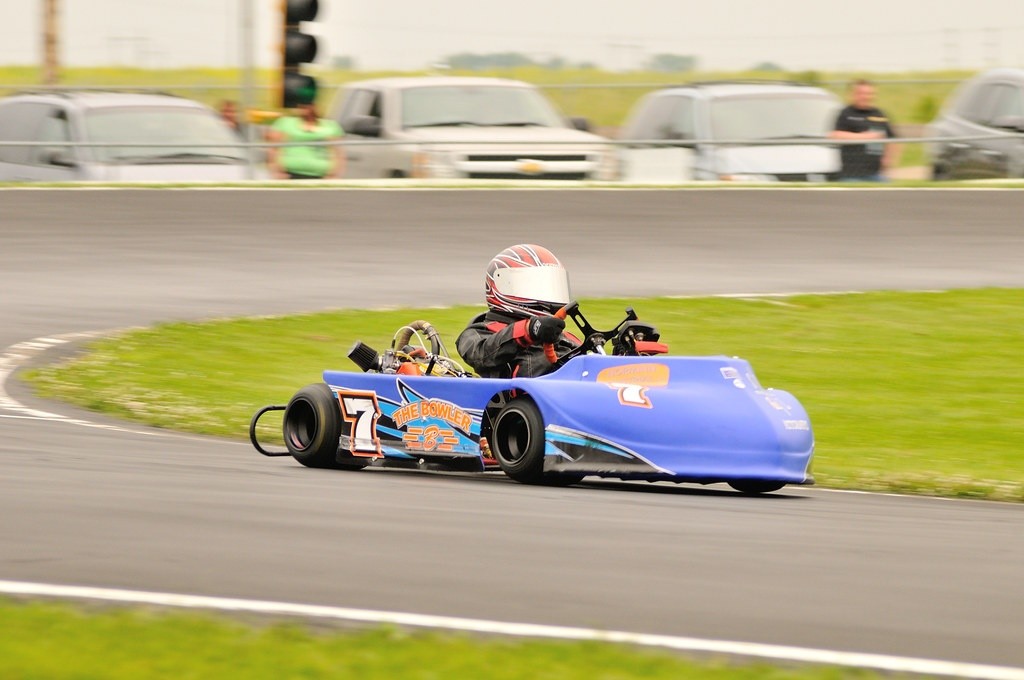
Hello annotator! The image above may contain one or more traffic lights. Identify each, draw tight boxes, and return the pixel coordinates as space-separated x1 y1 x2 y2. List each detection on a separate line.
271 1 321 110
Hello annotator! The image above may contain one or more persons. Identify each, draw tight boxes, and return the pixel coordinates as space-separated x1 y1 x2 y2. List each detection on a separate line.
832 77 897 183
455 244 590 377
264 79 345 179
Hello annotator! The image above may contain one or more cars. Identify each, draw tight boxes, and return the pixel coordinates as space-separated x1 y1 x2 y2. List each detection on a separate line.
598 82 854 185
2 90 278 185
917 66 1024 182
322 71 611 185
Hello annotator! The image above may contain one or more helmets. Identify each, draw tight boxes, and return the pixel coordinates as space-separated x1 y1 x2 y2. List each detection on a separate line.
486 244 570 318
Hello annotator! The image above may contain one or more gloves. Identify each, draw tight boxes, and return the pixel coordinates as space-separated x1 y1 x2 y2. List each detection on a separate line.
529 315 565 344
